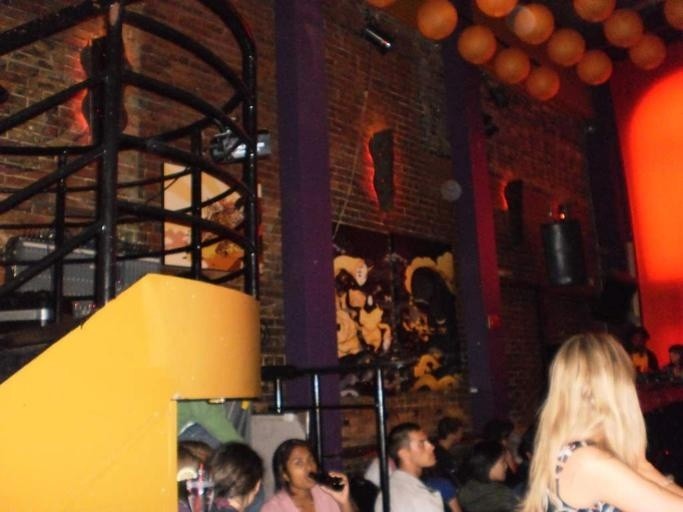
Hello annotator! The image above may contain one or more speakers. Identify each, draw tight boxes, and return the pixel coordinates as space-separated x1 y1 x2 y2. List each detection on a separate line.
541 218 585 287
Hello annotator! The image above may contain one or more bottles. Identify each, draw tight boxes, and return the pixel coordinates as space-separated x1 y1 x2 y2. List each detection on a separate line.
305 470 347 492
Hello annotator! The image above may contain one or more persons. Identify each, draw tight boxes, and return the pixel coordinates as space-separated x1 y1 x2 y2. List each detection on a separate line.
522 331 683 511
178 415 525 512
